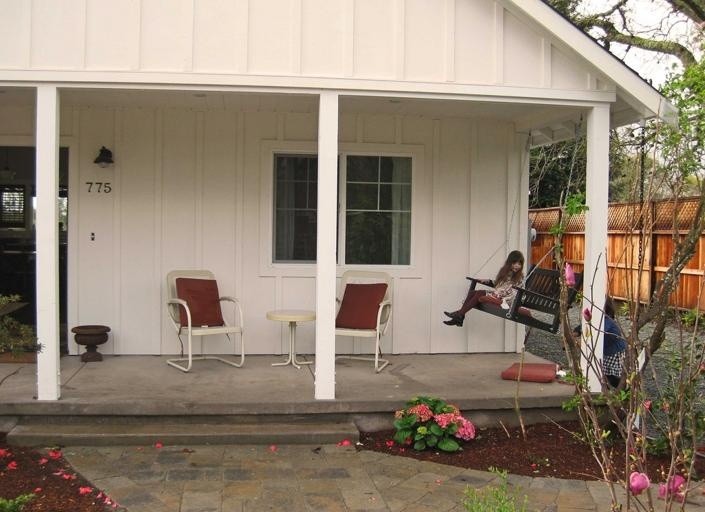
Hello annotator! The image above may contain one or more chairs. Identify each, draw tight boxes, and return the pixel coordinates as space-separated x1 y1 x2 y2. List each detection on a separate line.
330 268 398 375
164 267 245 373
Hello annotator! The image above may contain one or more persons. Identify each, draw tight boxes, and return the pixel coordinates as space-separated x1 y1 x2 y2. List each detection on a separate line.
572 296 627 390
443 251 531 327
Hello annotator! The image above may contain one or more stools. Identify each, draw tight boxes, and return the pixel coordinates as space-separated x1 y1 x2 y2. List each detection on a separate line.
69 324 111 363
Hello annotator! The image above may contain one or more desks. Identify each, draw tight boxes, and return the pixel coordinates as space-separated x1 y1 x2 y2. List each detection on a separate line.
266 309 316 369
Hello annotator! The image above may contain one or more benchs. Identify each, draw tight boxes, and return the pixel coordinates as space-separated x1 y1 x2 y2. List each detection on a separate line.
465 262 584 337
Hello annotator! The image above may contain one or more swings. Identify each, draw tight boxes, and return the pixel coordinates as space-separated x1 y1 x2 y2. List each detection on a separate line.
467 140 583 333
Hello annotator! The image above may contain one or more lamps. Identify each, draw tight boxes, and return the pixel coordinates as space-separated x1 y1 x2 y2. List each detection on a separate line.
91 144 115 169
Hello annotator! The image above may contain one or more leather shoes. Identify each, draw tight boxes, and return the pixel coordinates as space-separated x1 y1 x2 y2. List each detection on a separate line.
442 311 464 326
